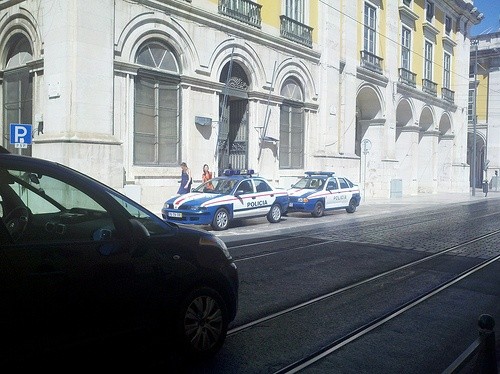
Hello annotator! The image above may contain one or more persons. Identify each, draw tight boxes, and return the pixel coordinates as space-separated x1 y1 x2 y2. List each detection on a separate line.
201 163 213 189
177 161 192 195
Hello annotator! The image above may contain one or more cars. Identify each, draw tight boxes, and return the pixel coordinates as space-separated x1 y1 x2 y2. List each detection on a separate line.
161 169 291 231
287 170 361 218
0 145 240 374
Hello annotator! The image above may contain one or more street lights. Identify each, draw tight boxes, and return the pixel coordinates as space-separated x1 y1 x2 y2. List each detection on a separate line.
470 38 480 197
